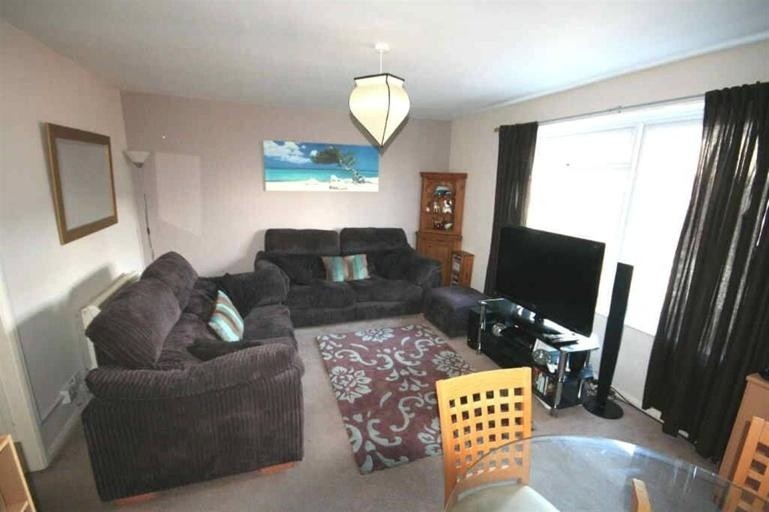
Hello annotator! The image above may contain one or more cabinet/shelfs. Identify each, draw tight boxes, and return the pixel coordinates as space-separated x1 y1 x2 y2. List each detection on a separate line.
449 250 474 286
415 231 463 288
419 172 467 235
711 370 768 507
467 298 600 418
0 433 37 512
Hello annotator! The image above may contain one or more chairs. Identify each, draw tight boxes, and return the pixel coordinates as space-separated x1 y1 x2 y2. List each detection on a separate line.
435 366 560 512
721 415 769 512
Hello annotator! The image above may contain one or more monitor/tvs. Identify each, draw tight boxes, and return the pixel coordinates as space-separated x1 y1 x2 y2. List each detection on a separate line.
497 226 605 337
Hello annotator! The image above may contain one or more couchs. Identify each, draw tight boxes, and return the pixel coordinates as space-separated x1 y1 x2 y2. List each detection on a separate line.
254 228 443 328
80 250 305 505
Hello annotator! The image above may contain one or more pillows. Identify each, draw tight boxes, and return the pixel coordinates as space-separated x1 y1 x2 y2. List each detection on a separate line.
206 272 262 320
187 338 262 362
207 289 245 342
320 254 371 283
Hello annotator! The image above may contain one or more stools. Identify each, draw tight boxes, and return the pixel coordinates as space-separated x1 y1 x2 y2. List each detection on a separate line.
423 286 487 339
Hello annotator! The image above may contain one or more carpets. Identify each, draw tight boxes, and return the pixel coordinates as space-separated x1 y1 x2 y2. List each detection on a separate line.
314 322 538 476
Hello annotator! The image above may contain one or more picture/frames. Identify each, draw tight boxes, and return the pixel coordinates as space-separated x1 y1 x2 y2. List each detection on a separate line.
45 122 118 245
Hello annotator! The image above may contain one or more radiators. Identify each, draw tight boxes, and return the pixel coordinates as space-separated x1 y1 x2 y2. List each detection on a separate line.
81 272 139 368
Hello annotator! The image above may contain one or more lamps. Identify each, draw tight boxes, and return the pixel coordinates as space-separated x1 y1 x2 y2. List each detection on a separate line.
349 46 411 148
124 150 155 262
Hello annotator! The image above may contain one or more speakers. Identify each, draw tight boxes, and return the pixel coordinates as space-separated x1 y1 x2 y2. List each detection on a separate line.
468 307 506 349
583 262 633 419
